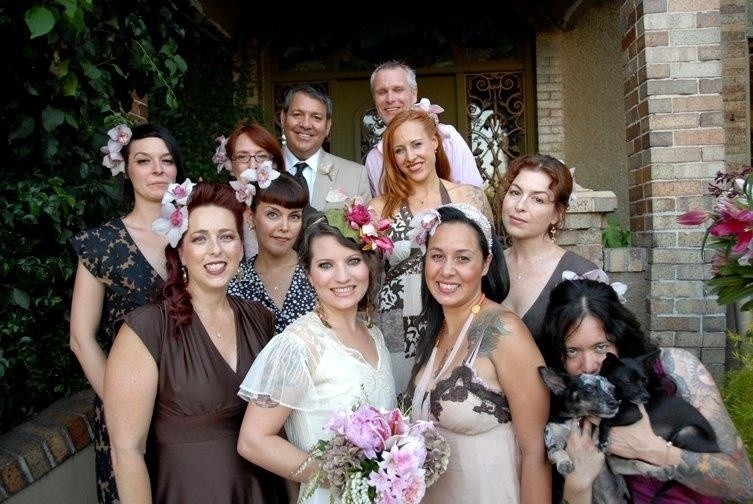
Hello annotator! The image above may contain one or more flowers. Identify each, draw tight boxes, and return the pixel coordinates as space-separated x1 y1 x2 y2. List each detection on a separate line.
228 160 280 206
404 208 441 256
675 163 752 312
413 97 444 124
99 123 132 176
150 177 196 249
323 194 394 254
288 381 450 503
211 133 231 174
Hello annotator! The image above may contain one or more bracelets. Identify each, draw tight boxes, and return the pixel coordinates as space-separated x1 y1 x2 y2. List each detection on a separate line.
660 440 674 468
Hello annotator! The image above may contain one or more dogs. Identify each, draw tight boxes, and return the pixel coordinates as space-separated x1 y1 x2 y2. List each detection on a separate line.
537 365 633 504
598 349 719 504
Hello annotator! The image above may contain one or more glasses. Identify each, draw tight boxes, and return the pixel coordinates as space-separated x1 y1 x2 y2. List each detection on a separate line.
230 153 274 164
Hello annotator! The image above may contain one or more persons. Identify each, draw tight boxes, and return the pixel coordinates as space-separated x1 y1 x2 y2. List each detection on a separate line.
367 108 498 415
536 270 753 504
276 84 374 222
105 182 300 504
212 122 287 264
68 122 185 504
221 167 321 337
499 152 602 367
363 59 484 201
233 196 398 504
404 201 554 504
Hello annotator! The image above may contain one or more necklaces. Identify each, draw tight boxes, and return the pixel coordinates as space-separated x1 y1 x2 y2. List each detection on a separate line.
432 345 455 378
504 243 554 280
272 285 282 292
192 300 231 338
411 194 425 206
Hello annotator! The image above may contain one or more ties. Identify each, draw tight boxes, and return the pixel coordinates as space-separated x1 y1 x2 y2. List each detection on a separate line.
294 162 308 188
